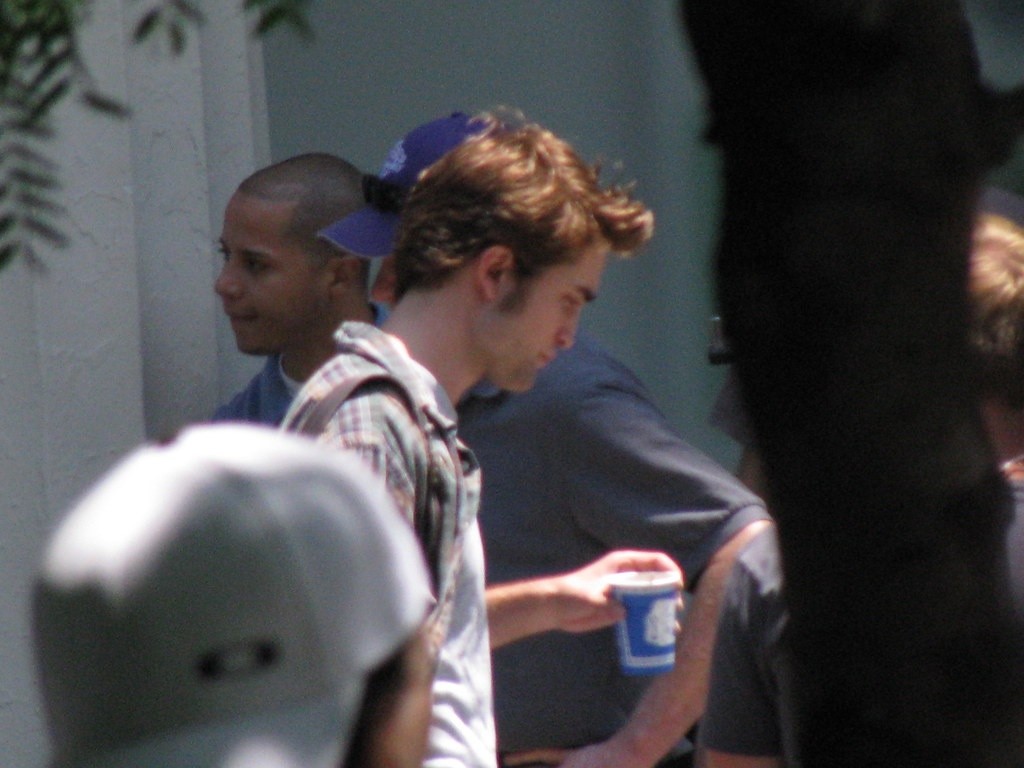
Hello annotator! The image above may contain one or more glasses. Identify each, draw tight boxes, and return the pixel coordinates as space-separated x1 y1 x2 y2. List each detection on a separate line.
362 175 409 212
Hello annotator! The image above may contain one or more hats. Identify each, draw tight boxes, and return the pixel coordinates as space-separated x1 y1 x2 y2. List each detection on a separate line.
316 112 497 255
32 422 430 767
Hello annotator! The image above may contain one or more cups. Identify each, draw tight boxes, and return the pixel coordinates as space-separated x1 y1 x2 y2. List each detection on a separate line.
605 570 680 675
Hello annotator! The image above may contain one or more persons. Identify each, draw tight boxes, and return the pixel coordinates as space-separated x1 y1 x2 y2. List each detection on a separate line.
28 111 774 768
683 210 1024 765
